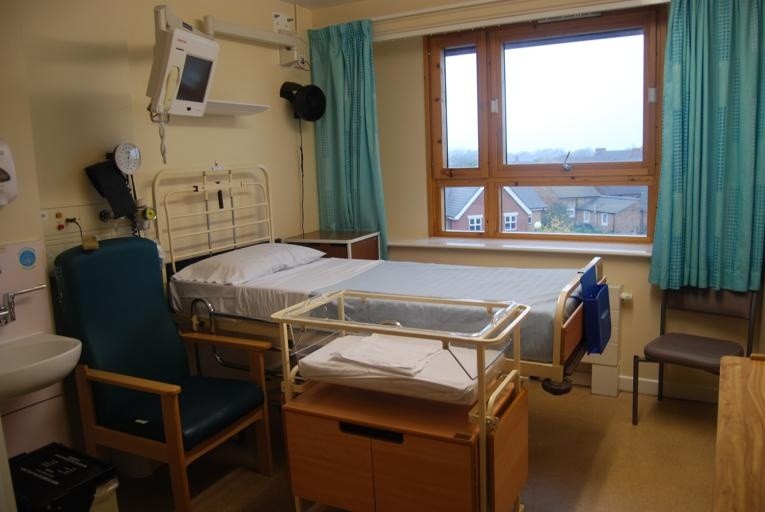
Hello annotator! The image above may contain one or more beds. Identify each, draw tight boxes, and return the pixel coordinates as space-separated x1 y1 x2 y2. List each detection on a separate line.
152 164 610 451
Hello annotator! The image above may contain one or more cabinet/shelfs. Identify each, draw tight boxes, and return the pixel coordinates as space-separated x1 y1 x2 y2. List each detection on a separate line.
280 379 530 512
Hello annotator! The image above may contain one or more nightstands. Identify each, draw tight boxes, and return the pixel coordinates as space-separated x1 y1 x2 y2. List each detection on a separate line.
287 231 382 260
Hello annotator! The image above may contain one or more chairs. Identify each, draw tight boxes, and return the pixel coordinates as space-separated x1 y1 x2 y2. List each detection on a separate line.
52 231 275 510
633 283 758 425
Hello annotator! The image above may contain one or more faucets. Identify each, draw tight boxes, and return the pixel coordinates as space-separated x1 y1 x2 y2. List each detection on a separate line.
0 303 14 323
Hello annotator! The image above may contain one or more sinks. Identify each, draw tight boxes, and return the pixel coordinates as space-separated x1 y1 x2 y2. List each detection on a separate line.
0 331 82 396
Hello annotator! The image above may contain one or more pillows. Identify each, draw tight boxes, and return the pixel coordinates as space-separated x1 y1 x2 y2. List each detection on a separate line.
171 243 329 285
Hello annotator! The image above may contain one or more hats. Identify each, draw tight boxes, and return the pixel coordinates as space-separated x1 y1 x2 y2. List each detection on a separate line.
280 80 326 121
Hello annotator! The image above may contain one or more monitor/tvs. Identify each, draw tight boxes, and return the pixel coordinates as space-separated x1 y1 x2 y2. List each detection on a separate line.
151 28 220 117
